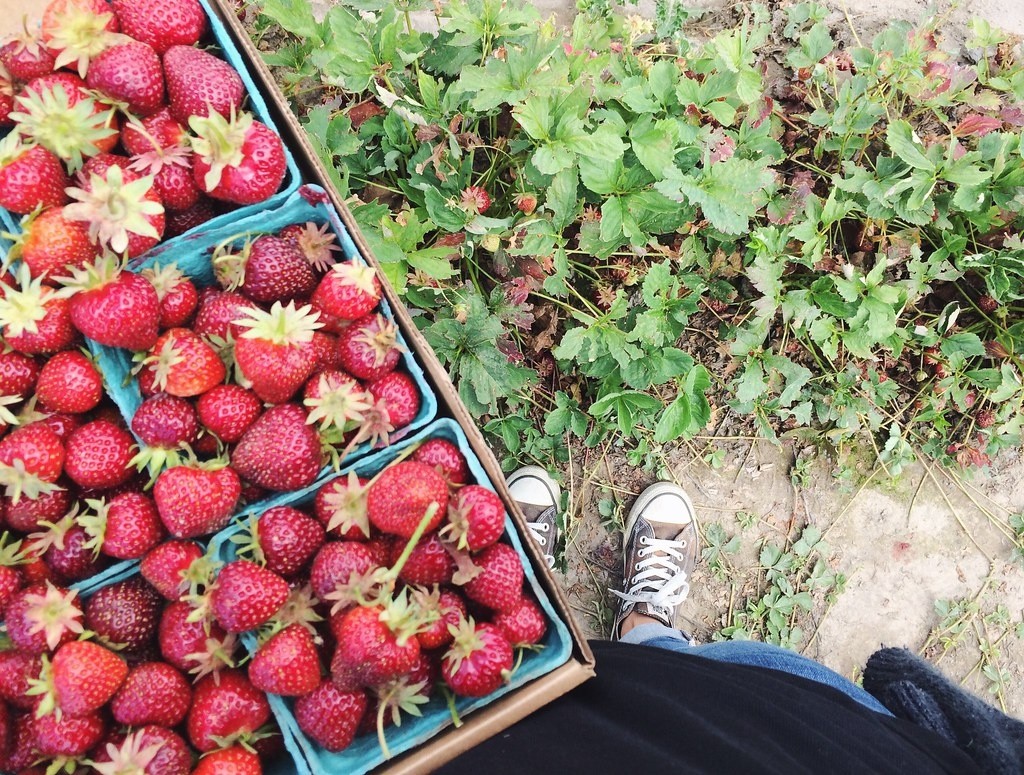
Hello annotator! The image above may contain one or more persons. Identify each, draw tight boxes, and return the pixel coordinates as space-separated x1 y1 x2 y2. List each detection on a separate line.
429 464 1024 775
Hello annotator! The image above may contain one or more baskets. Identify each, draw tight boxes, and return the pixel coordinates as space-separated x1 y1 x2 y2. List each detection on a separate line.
1 0 574 775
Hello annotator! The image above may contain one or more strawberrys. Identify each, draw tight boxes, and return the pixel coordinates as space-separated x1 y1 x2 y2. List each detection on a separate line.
915 349 995 429
0 0 543 775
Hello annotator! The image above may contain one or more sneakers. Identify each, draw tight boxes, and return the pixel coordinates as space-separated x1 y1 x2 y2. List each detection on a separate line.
507 464 560 573
611 482 698 647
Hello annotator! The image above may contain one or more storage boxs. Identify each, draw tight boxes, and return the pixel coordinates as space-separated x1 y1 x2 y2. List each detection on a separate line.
1 0 598 775
